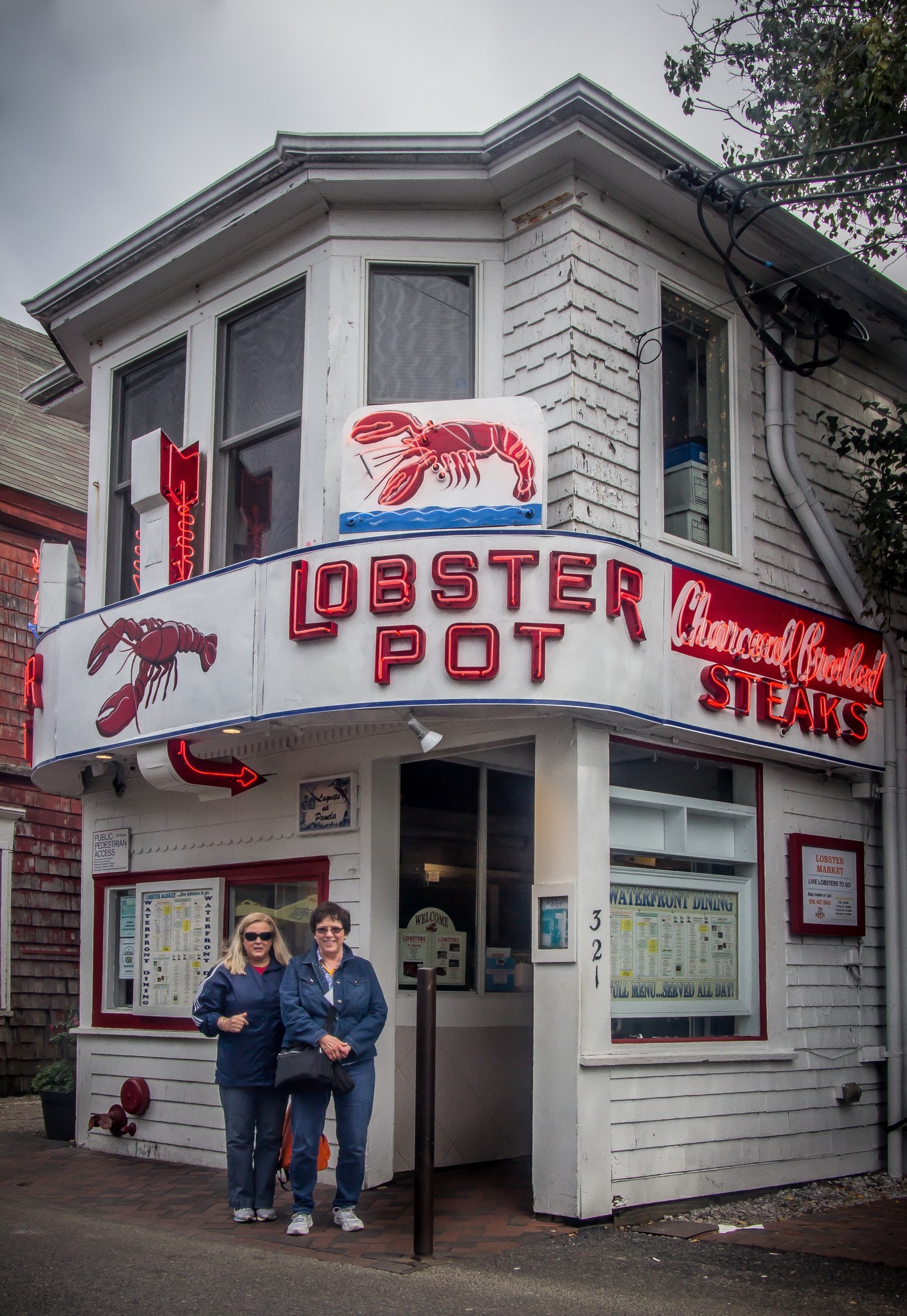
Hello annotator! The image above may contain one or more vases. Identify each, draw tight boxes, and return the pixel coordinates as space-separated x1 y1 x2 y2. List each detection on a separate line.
40 1090 76 1141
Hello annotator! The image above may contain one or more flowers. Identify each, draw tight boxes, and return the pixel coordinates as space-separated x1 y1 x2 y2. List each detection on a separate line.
30 1008 80 1093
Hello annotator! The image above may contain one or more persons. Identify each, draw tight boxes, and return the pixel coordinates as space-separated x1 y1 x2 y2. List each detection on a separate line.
191 912 292 1223
550 923 564 948
274 901 389 1236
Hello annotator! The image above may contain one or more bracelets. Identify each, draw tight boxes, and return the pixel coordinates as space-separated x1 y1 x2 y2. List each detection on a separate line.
218 1017 228 1032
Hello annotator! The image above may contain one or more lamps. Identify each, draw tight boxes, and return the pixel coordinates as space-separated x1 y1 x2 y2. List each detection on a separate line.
408 711 443 753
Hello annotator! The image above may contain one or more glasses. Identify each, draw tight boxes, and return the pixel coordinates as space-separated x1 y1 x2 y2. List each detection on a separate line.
244 931 273 941
315 926 344 934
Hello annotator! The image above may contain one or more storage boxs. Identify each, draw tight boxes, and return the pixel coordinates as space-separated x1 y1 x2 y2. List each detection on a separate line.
664 443 710 547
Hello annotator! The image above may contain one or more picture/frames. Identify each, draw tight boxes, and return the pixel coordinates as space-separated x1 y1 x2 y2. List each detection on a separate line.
295 773 359 837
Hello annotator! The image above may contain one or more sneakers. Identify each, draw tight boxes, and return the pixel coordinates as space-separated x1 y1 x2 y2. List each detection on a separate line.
287 1212 313 1235
257 1208 277 1221
334 1206 364 1232
233 1207 256 1222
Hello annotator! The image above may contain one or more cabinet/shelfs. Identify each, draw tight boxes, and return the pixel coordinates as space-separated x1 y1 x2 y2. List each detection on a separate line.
609 786 758 864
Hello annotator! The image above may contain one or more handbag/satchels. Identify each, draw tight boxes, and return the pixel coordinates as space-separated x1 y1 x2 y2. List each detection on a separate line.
273 1044 355 1094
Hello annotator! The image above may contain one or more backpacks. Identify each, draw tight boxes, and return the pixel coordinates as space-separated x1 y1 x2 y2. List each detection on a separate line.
277 1101 330 1192
